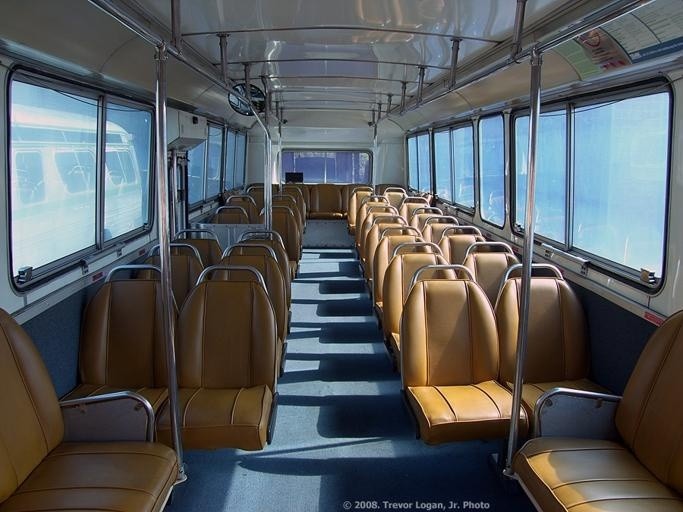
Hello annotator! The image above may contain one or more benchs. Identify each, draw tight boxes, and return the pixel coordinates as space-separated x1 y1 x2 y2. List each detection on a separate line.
501 308 682 512
1 304 186 511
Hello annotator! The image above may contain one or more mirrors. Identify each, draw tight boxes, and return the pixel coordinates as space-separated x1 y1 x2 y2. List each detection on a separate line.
228 82 266 117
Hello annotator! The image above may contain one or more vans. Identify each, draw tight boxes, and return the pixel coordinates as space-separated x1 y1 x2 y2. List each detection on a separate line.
9 101 146 278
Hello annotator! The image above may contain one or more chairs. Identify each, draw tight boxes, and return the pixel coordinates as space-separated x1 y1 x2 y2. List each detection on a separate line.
56 182 620 447
